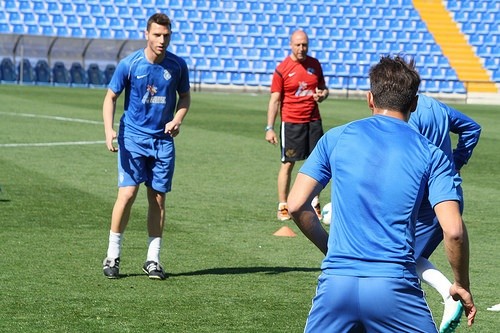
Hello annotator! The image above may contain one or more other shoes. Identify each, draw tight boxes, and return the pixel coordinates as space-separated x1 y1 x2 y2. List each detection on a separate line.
277 202 290 219
311 197 322 219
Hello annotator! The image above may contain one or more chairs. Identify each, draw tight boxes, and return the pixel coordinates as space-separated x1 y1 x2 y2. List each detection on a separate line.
0 0 500 93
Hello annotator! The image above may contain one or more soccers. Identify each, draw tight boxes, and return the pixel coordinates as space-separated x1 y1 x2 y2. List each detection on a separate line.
321 202 332 225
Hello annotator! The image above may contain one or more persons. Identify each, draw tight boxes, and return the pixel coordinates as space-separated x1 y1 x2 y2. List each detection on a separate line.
102 13 190 279
321 90 482 333
265 30 329 222
287 56 477 333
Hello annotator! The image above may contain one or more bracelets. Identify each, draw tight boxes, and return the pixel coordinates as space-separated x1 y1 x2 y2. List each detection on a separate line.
265 126 274 131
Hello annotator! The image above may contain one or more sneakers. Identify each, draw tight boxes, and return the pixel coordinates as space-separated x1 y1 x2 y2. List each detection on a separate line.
103 256 120 278
439 294 464 332
142 260 165 279
